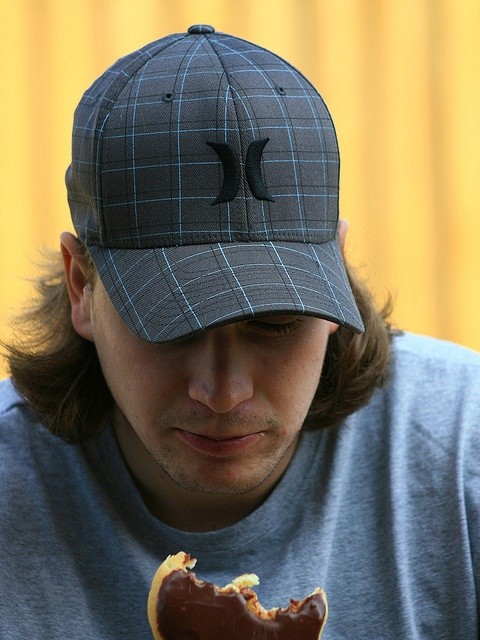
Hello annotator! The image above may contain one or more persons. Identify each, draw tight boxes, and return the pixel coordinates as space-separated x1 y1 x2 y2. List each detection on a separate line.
0 24 478 636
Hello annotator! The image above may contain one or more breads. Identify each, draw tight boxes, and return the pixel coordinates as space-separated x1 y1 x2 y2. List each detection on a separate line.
146 550 328 640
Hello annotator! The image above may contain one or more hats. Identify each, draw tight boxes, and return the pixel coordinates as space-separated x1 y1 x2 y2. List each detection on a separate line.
63 25 365 343
